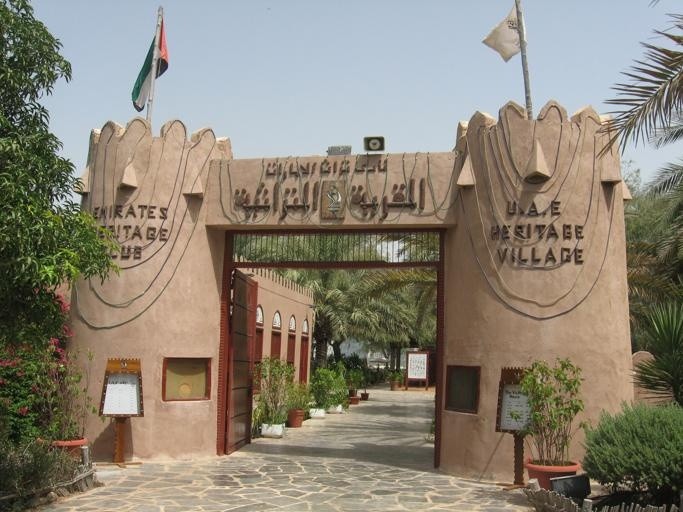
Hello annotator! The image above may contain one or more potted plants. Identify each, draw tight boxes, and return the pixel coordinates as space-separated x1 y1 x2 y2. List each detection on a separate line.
510 355 588 491
251 357 368 442
387 369 403 391
33 327 98 465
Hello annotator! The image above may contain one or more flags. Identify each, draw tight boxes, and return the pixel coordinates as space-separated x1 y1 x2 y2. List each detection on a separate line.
481 3 527 61
131 13 168 112
